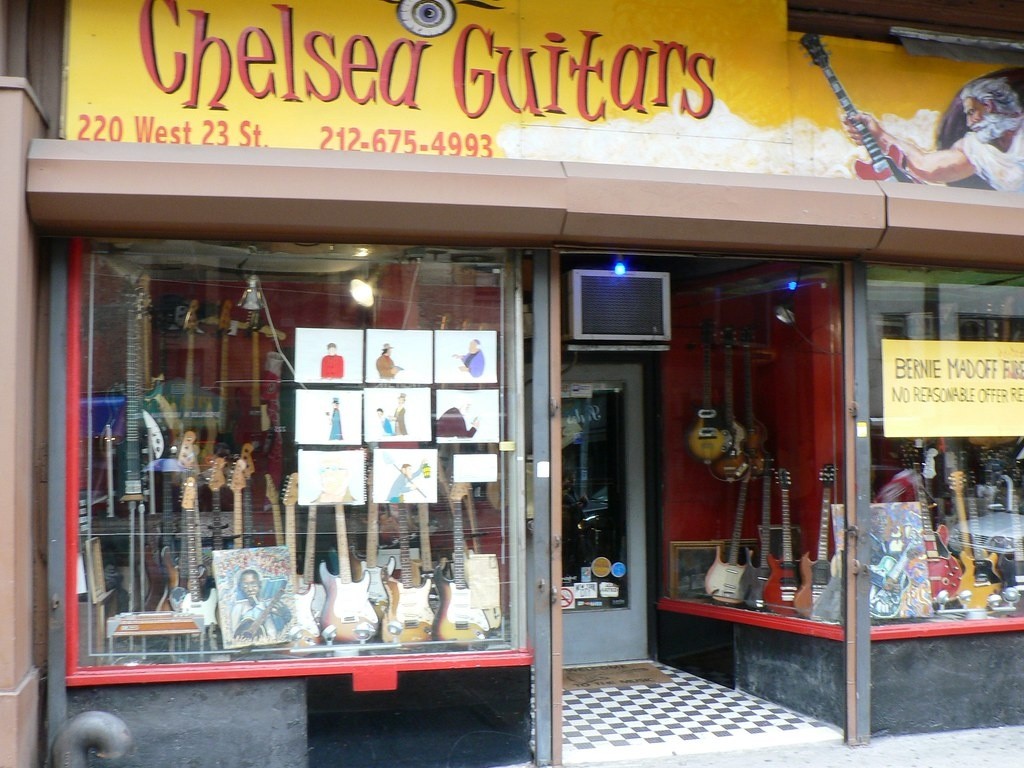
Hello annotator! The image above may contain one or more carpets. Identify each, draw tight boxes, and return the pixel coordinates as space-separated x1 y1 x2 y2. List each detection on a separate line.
563 662 675 691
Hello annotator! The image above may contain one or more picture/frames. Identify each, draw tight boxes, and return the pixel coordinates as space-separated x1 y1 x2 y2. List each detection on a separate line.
712 538 760 569
757 525 802 561
669 541 725 601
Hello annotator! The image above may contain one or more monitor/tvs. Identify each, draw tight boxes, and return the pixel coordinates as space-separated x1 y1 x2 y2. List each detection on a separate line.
568 268 673 342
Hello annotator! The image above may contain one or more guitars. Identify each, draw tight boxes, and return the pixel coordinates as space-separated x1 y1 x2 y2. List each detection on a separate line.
82 269 507 657
683 290 1024 622
